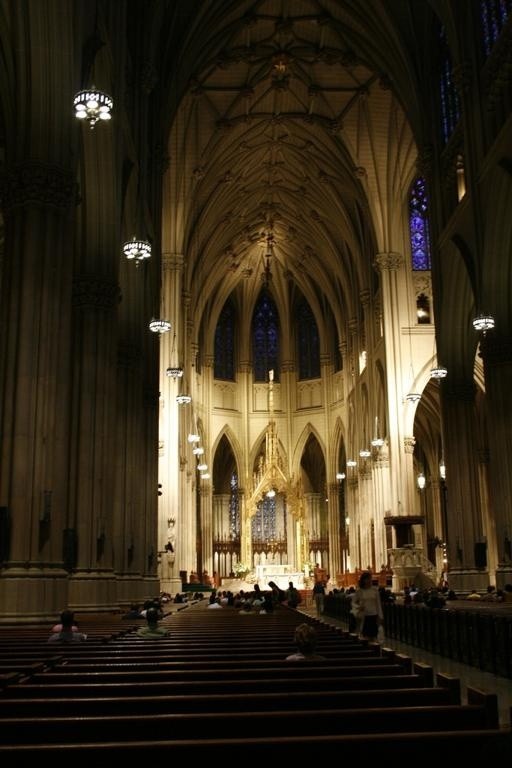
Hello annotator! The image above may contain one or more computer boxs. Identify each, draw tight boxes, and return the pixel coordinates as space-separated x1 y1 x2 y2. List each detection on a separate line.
164 540 176 554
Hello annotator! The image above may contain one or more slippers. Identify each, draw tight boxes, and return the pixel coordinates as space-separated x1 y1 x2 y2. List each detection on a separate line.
276 587 286 602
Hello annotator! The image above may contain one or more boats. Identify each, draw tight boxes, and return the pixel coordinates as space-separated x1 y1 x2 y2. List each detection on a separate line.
474 542 487 568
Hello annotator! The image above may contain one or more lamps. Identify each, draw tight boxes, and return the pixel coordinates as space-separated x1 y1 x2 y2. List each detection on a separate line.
149 315 172 334
123 233 153 265
165 365 184 380
178 394 190 406
393 99 496 405
73 86 113 130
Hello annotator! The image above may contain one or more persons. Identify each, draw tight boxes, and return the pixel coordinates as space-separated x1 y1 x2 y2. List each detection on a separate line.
137 608 169 637
351 572 386 647
283 623 327 660
312 572 512 615
48 616 84 642
123 604 145 619
140 600 162 617
173 581 301 614
49 609 80 632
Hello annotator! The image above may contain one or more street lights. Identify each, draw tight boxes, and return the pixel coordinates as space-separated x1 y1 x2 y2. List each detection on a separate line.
349 606 365 634
377 625 385 643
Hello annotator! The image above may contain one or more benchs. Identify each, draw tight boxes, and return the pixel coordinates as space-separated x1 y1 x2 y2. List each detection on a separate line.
0 590 512 768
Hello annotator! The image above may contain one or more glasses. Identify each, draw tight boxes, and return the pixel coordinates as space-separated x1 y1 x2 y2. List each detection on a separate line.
393 99 496 405
